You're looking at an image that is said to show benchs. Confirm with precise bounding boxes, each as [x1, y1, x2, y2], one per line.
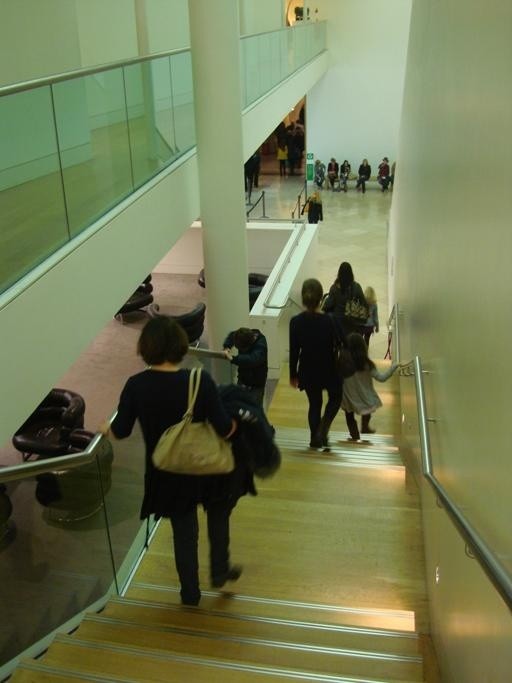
[315, 171, 393, 191]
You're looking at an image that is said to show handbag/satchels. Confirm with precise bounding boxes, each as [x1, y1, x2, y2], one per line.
[150, 367, 236, 475]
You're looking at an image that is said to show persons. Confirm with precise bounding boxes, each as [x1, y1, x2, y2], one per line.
[327, 158, 340, 191]
[362, 287, 379, 347]
[244, 157, 255, 197]
[300, 192, 323, 223]
[276, 121, 305, 176]
[340, 160, 351, 193]
[253, 153, 259, 188]
[314, 160, 326, 191]
[355, 159, 372, 194]
[96, 315, 243, 608]
[288, 278, 369, 448]
[341, 332, 401, 440]
[222, 327, 268, 407]
[324, 262, 368, 335]
[377, 157, 391, 193]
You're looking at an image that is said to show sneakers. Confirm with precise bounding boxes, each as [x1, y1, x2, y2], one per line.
[209, 565, 243, 589]
[360, 426, 376, 434]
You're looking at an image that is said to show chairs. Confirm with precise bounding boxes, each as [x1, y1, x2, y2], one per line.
[0, 387, 115, 552]
[118, 273, 269, 346]
